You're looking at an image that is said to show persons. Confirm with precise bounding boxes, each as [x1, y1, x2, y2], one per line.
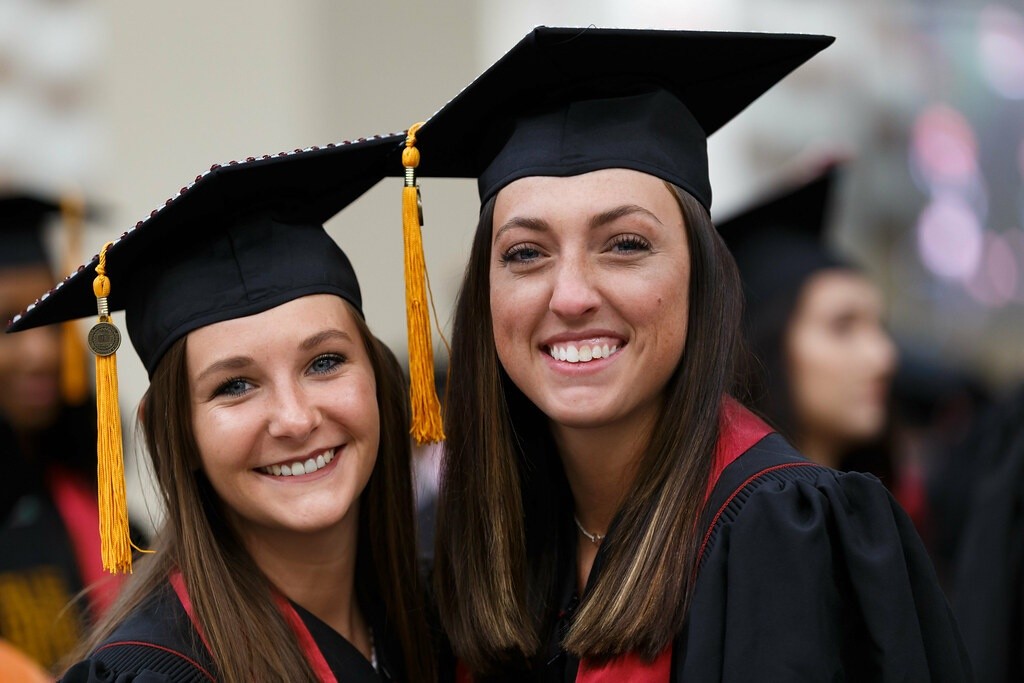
[1, 183, 154, 682]
[713, 160, 897, 470]
[383, 26, 976, 683]
[7, 129, 438, 683]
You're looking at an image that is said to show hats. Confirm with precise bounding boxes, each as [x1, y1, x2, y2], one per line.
[399, 24, 836, 451]
[711, 175, 854, 309]
[2, 191, 98, 407]
[4, 132, 409, 577]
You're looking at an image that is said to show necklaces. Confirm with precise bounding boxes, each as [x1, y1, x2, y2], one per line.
[569, 509, 617, 547]
[363, 616, 382, 677]
